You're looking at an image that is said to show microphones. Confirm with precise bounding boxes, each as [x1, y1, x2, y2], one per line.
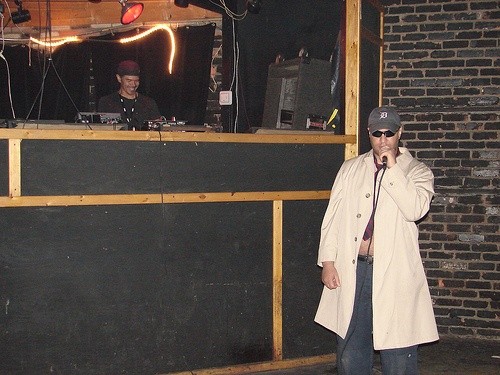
[381, 156, 388, 170]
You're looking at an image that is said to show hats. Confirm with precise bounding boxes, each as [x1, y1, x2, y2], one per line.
[368, 107, 401, 134]
[118, 60, 141, 75]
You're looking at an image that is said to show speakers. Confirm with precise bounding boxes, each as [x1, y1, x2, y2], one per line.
[261, 57, 333, 130]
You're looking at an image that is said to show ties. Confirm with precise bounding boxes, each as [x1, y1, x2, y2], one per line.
[363, 147, 401, 241]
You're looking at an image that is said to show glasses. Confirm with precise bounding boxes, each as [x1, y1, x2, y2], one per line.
[371, 130, 396, 138]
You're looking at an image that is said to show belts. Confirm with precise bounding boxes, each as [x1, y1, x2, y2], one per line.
[358, 255, 373, 265]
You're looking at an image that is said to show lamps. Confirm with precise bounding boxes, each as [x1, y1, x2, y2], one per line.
[11, 0, 31, 24]
[119, 0, 144, 25]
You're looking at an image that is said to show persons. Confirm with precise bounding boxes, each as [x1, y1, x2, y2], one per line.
[97, 61, 160, 131]
[314, 106, 439, 373]
